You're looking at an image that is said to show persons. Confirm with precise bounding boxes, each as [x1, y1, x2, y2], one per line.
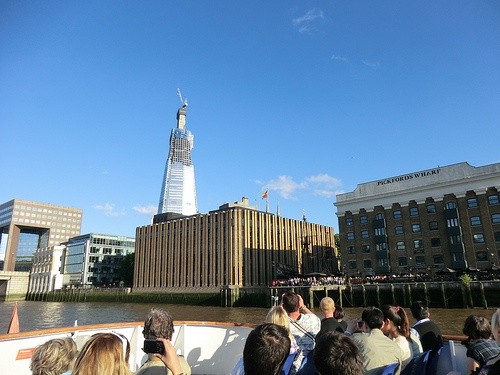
[28, 306, 191, 375]
[228, 290, 500, 375]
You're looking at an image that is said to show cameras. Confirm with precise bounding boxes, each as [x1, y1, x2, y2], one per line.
[144, 339, 164, 354]
